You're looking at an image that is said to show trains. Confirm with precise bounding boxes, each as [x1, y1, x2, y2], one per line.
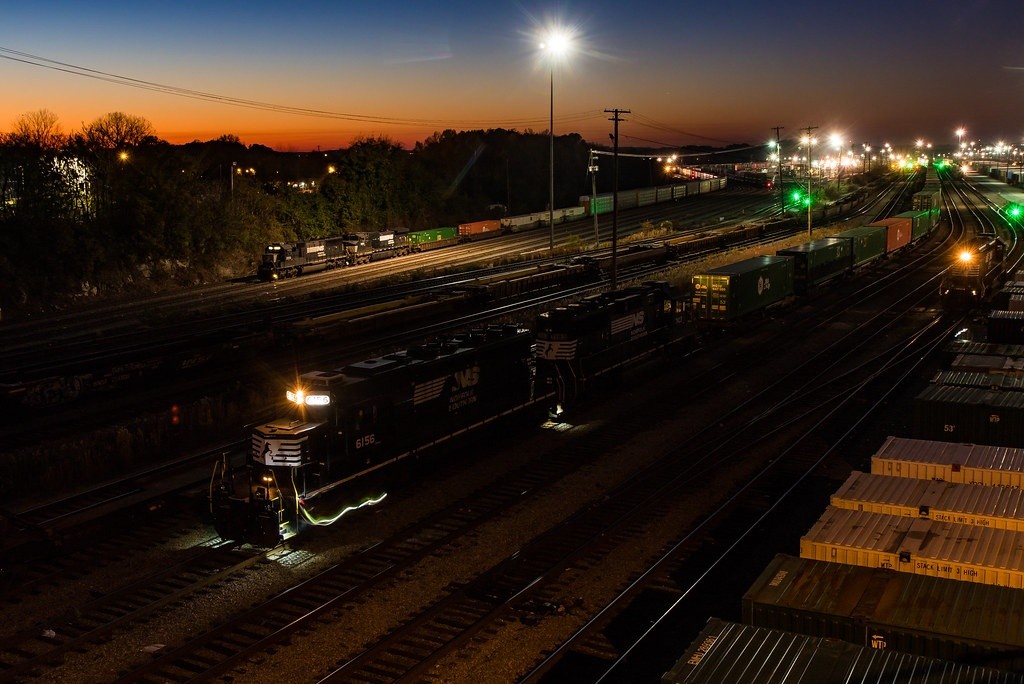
[255, 164, 731, 283]
[210, 163, 940, 526]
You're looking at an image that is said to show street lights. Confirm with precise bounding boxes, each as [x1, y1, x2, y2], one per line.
[533, 22, 1023, 253]
